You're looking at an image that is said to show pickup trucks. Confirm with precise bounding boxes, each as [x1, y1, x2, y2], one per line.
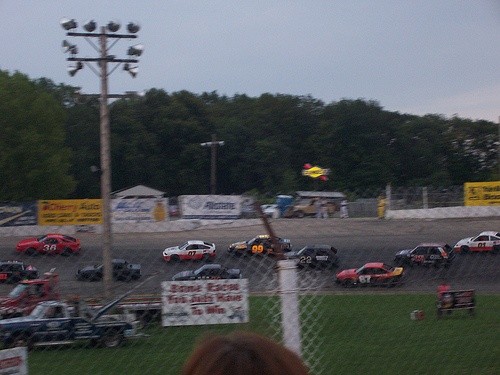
[0, 268, 81, 319]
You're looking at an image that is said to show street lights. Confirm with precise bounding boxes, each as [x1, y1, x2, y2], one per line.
[200, 140, 226, 195]
[59, 17, 145, 317]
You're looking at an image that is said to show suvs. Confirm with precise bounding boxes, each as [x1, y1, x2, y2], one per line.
[393, 242, 456, 269]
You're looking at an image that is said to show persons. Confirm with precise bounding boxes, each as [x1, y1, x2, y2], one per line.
[376, 194, 385, 218]
[182, 329, 312, 375]
[436, 281, 450, 301]
[339, 200, 348, 218]
[319, 199, 329, 218]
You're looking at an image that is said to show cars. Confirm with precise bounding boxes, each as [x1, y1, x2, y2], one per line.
[334, 262, 406, 289]
[282, 244, 339, 271]
[0, 299, 135, 352]
[171, 264, 243, 281]
[453, 230, 500, 256]
[289, 198, 335, 219]
[16, 233, 82, 257]
[163, 240, 217, 265]
[227, 234, 293, 260]
[75, 259, 143, 284]
[0, 259, 40, 284]
